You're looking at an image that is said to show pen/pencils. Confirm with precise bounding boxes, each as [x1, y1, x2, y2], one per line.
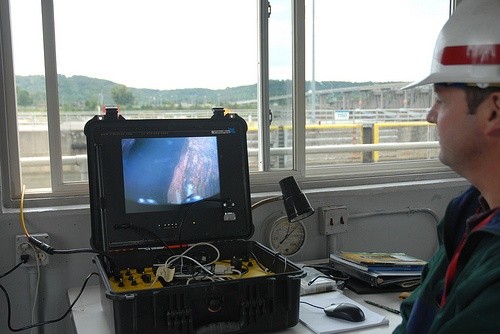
[363, 299, 399, 314]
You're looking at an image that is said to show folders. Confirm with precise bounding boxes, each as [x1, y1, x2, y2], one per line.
[329, 258, 426, 286]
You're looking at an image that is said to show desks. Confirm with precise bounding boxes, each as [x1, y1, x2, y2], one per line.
[67, 257, 408, 334]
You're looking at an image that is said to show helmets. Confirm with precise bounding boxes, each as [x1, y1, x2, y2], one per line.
[401, 0, 500, 93]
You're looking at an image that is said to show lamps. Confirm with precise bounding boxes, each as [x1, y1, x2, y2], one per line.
[251, 176, 316, 223]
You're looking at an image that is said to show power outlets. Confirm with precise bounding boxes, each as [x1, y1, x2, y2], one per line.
[16, 233, 51, 268]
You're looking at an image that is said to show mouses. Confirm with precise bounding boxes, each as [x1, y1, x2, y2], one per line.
[324, 303, 365, 322]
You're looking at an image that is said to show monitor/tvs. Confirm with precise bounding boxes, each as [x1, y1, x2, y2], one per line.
[83, 106, 254, 251]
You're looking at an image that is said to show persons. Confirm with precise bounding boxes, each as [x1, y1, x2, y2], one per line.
[392, 0, 500, 334]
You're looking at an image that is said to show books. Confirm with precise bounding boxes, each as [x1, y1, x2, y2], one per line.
[331, 249, 426, 271]
